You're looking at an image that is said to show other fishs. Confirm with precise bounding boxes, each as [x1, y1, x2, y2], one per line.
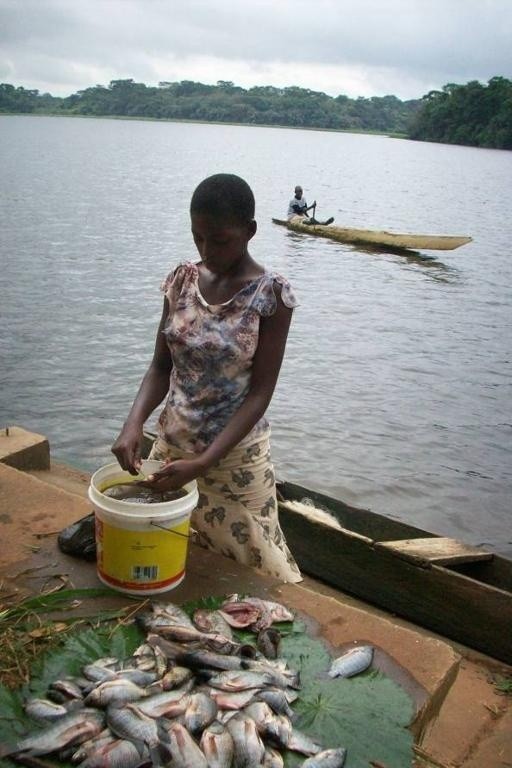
[99, 457, 188, 504]
[1, 592, 349, 767]
[325, 644, 375, 679]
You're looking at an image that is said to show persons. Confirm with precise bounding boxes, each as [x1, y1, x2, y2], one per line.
[111, 174, 303, 585]
[288, 185, 334, 226]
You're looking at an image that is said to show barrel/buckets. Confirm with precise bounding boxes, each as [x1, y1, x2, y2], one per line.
[86, 460, 200, 597]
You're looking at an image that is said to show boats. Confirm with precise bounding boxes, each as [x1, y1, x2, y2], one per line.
[273, 217, 473, 250]
[142, 433, 512, 661]
